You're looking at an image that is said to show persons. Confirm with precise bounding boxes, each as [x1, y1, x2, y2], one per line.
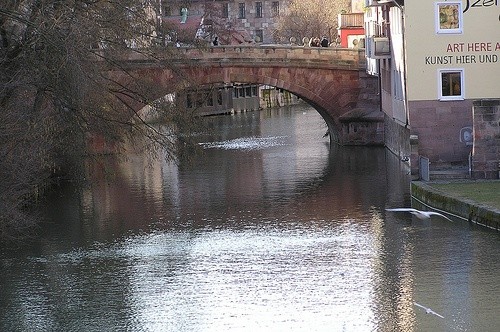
[336, 35, 342, 44]
[321, 36, 328, 47]
[310, 37, 321, 47]
[213, 37, 218, 45]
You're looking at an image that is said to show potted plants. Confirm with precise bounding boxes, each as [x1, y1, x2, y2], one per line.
[374, 33, 389, 53]
